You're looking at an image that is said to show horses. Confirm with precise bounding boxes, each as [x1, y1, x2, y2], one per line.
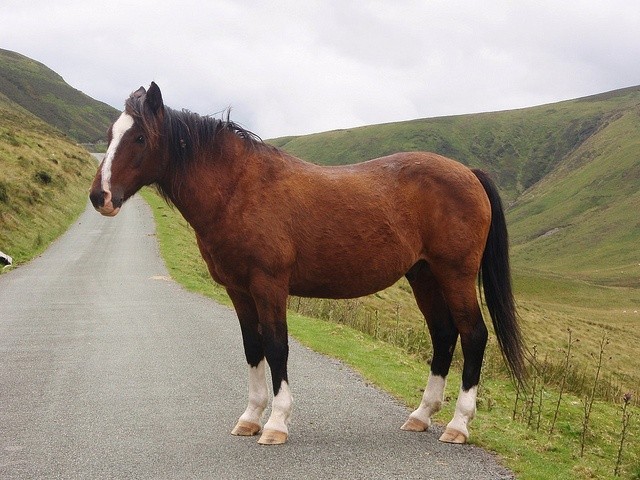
[86, 79, 544, 445]
